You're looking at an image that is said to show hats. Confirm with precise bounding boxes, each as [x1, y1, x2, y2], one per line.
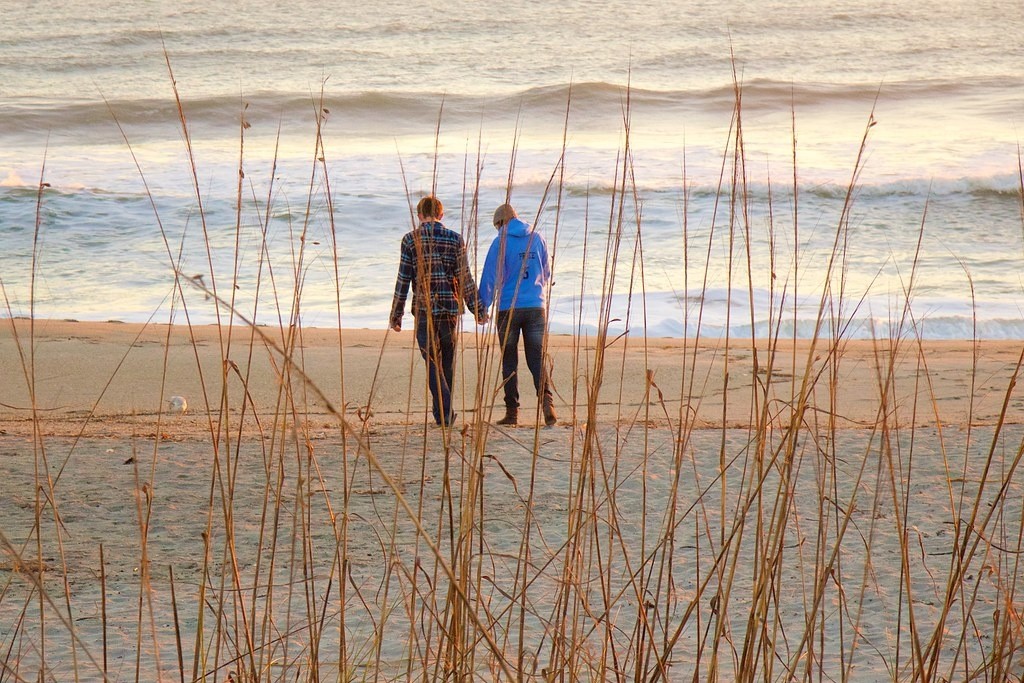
[493, 204, 517, 226]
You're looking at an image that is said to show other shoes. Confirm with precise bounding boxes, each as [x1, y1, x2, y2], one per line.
[436, 413, 450, 426]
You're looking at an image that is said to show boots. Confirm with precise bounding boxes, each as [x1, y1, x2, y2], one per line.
[496, 407, 519, 425]
[540, 392, 557, 425]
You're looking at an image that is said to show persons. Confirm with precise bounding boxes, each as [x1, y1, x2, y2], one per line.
[475, 205, 559, 425]
[389, 197, 489, 426]
